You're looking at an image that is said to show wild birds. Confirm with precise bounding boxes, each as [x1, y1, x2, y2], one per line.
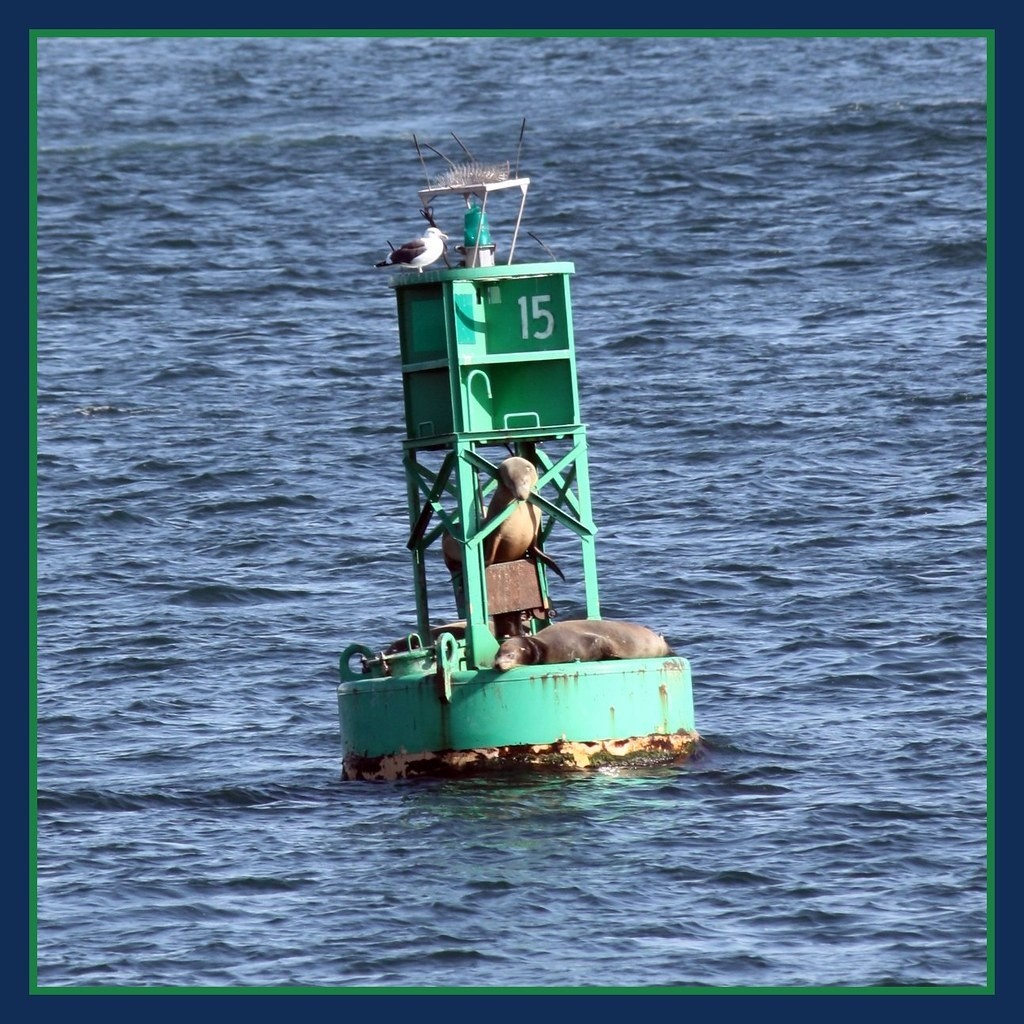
[373, 227, 448, 274]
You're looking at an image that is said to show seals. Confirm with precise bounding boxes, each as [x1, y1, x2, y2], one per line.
[494, 619, 674, 673]
[442, 456, 566, 583]
